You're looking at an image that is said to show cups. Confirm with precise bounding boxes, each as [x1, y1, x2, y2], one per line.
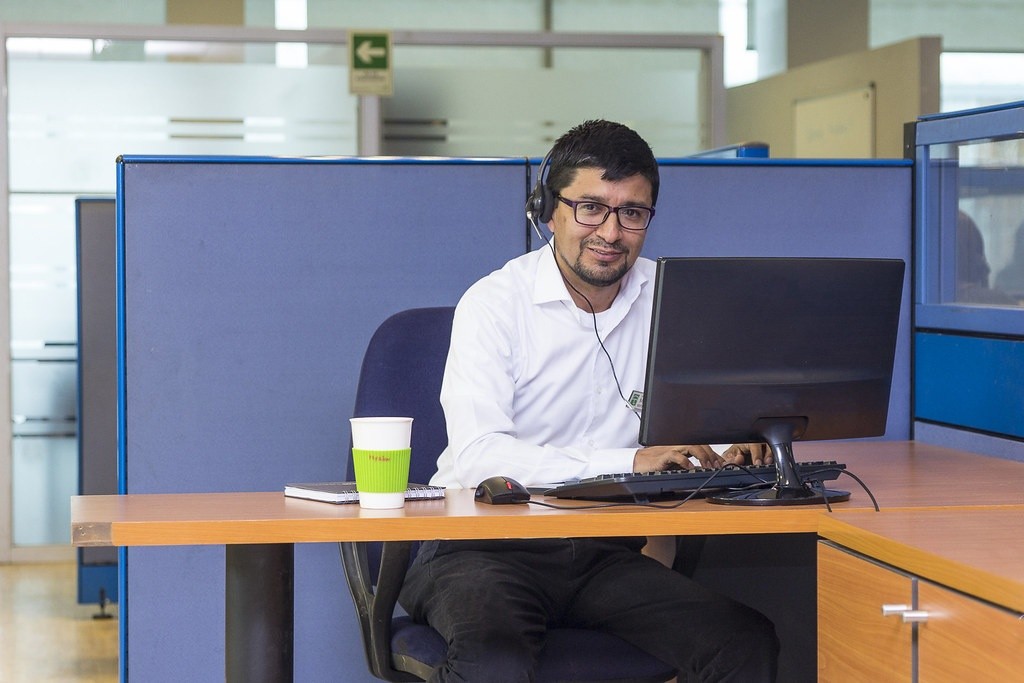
[349, 417, 414, 509]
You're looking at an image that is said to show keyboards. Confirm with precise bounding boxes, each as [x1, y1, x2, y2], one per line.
[544, 459, 846, 502]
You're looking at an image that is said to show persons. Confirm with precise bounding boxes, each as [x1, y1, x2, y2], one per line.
[955, 208, 1023, 304]
[396, 116, 774, 681]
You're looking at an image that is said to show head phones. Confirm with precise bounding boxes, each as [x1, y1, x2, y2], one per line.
[525, 149, 556, 239]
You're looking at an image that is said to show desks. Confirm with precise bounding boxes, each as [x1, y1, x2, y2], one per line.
[71, 440, 1024, 683]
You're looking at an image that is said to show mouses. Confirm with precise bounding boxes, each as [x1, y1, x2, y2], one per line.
[474, 476, 530, 505]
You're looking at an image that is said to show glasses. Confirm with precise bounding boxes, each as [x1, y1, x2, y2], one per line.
[557, 194, 655, 230]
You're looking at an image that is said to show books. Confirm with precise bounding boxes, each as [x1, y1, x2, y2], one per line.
[284, 479, 446, 506]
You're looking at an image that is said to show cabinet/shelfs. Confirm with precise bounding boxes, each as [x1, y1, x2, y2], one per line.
[817, 508, 1024, 682]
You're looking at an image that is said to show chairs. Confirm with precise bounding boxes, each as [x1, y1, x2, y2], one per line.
[340, 305, 459, 683]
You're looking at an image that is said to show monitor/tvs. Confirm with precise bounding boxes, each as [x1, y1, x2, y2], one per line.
[638, 258, 906, 505]
